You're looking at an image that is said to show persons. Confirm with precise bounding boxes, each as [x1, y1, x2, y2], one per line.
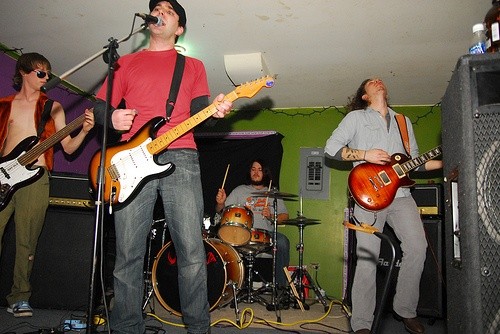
[0, 52, 95, 317]
[216, 160, 289, 301]
[323, 78, 442, 334]
[93, 0, 233, 334]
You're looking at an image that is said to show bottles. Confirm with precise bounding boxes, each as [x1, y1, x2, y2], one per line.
[484, 0, 500, 52]
[469, 23, 488, 55]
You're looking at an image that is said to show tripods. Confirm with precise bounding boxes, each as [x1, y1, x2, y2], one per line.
[241, 198, 330, 311]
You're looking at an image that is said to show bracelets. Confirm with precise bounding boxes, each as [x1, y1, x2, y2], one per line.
[364, 150, 367, 161]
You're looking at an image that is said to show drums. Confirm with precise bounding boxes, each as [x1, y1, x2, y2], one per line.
[151, 237, 245, 318]
[215, 203, 255, 247]
[248, 228, 273, 247]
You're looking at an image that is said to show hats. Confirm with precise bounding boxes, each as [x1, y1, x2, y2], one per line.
[149, 0, 186, 28]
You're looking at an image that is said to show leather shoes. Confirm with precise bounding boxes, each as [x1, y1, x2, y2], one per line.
[355, 329, 371, 334]
[393, 311, 425, 334]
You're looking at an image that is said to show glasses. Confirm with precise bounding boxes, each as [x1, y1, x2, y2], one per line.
[34, 70, 52, 78]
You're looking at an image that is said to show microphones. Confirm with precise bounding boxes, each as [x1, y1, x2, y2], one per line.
[135, 12, 163, 27]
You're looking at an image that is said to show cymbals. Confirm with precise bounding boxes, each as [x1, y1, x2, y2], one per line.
[279, 216, 322, 226]
[251, 191, 299, 202]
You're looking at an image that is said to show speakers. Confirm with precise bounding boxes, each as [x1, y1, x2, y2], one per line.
[0, 206, 108, 313]
[347, 217, 445, 320]
[440, 50, 500, 334]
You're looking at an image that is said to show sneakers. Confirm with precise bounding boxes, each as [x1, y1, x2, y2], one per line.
[7, 301, 33, 317]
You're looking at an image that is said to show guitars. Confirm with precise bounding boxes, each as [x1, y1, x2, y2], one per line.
[347, 144, 442, 214]
[0, 107, 95, 212]
[87, 74, 275, 212]
